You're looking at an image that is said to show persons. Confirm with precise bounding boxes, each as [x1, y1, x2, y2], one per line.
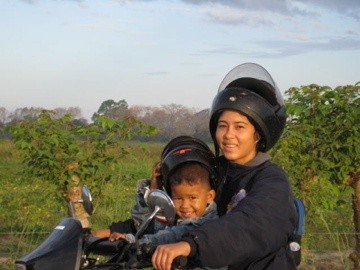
[108, 162, 228, 270]
[90, 102, 299, 270]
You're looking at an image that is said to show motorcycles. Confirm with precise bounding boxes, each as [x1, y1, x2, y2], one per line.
[15, 183, 177, 270]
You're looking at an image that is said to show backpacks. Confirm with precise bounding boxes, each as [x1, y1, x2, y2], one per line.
[289, 198, 304, 266]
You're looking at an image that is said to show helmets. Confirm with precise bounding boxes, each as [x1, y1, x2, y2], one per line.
[160, 136, 219, 196]
[209, 63, 286, 153]
